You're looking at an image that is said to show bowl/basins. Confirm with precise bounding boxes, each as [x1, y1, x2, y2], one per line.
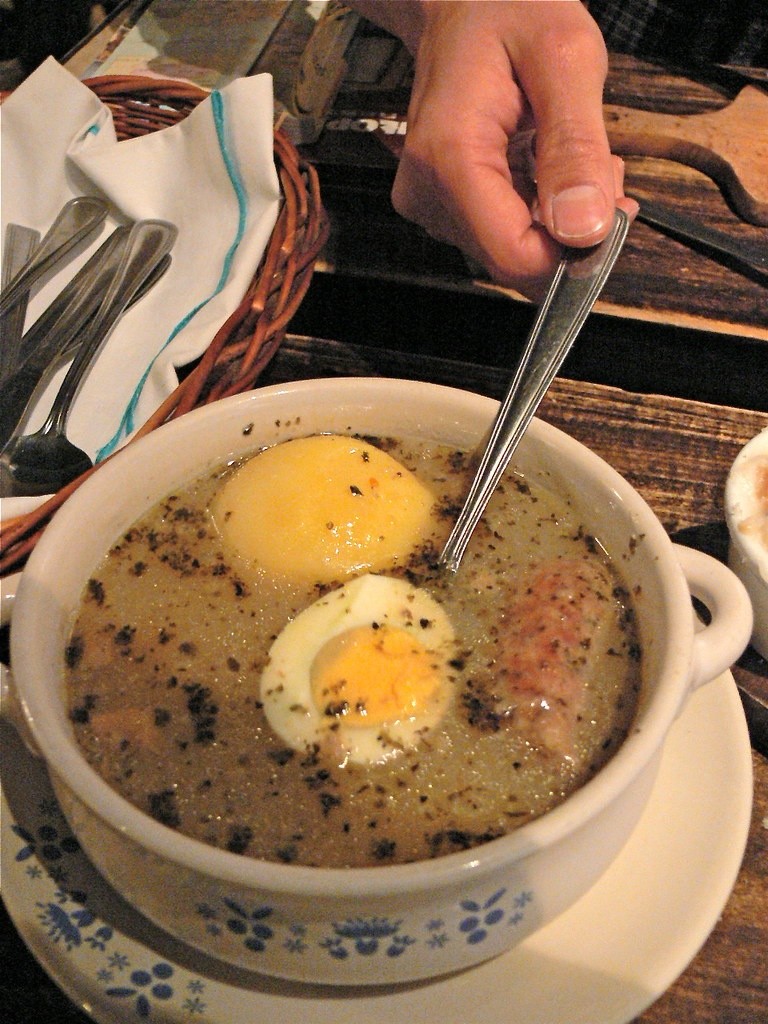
[0, 376, 754, 983]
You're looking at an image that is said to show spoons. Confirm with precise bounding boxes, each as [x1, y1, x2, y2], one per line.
[0, 219, 177, 500]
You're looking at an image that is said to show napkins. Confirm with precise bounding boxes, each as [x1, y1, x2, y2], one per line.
[0, 56, 283, 528]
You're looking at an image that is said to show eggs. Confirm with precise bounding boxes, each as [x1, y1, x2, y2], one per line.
[257, 570, 458, 768]
[207, 436, 442, 589]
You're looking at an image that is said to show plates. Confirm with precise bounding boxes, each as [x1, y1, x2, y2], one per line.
[0, 614, 752, 1024]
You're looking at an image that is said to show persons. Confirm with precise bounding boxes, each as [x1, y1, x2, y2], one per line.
[351, 1, 768, 310]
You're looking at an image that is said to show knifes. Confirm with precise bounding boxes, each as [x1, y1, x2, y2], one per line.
[0, 226, 137, 456]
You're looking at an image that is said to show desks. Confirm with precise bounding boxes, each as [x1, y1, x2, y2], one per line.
[0, 0, 768, 1024]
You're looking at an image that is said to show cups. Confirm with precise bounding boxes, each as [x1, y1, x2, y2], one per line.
[724, 428, 767, 661]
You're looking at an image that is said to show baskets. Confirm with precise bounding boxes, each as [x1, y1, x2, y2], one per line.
[0, 74, 331, 580]
